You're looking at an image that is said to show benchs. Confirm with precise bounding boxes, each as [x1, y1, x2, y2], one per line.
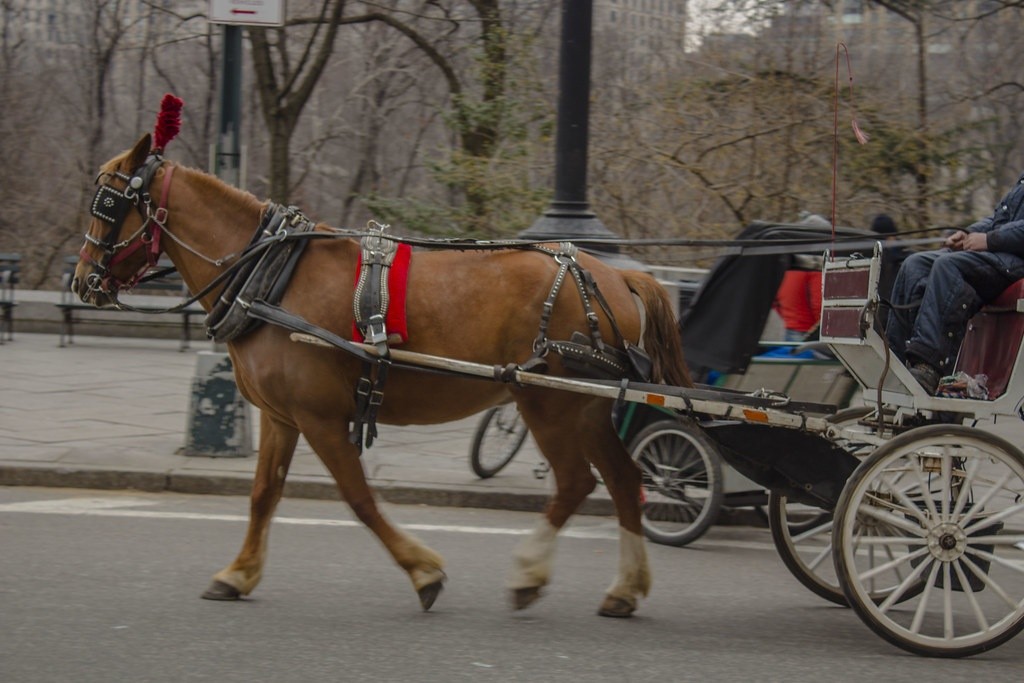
[952, 277, 1024, 399]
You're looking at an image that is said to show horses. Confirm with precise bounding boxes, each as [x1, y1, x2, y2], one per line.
[69, 133, 710, 619]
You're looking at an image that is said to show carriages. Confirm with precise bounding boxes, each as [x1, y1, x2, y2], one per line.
[70, 94, 1024, 661]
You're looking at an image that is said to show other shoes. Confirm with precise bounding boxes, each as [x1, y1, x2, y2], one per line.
[908, 362, 940, 397]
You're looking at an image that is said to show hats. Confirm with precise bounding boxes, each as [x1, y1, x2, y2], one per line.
[873, 214, 898, 236]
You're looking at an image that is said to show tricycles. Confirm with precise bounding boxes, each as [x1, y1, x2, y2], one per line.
[470, 394, 834, 545]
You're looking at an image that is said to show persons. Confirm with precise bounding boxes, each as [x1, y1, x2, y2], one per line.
[882, 173, 1024, 396]
[869, 213, 898, 240]
